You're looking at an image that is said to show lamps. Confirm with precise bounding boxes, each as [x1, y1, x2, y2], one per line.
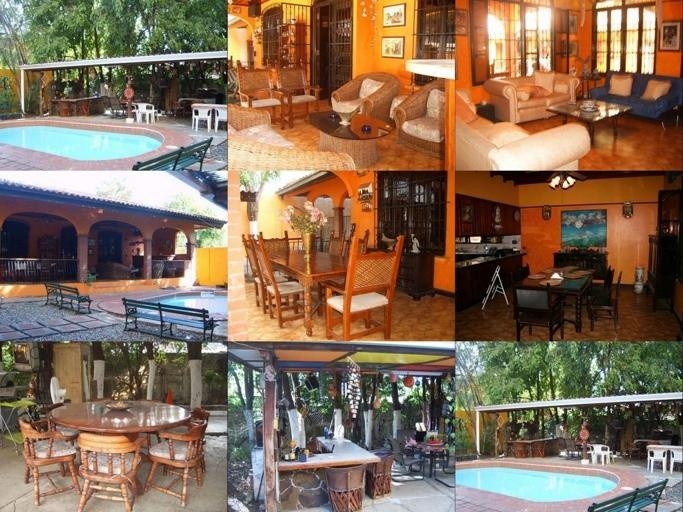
[548, 175, 579, 192]
[541, 204, 552, 222]
[622, 199, 633, 219]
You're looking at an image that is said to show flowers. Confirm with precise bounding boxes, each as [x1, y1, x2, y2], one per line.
[281, 199, 330, 237]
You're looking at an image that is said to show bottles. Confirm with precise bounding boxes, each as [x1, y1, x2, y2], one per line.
[327, 429, 332, 444]
[323, 424, 329, 444]
[94, 90, 98, 99]
[291, 19, 296, 25]
[581, 64, 587, 80]
[283, 446, 299, 461]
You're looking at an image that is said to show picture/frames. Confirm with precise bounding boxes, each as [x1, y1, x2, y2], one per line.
[381, 2, 406, 28]
[657, 21, 682, 54]
[380, 35, 405, 60]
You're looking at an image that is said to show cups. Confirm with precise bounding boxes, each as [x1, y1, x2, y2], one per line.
[276, 19, 280, 25]
[286, 19, 290, 24]
[299, 453, 305, 462]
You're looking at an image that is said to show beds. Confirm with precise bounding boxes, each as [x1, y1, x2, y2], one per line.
[302, 108, 394, 178]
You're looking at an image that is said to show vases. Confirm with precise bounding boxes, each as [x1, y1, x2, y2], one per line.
[300, 228, 316, 260]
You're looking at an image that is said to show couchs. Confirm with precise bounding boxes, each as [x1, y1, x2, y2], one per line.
[227, 101, 356, 173]
[590, 68, 682, 131]
[482, 69, 582, 126]
[455, 89, 593, 174]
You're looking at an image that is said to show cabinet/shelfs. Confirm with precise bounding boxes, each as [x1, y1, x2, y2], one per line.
[259, 6, 308, 77]
[455, 190, 522, 241]
[378, 243, 437, 303]
[644, 230, 683, 314]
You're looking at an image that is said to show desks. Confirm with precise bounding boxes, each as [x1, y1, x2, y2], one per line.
[274, 431, 383, 471]
[506, 435, 556, 458]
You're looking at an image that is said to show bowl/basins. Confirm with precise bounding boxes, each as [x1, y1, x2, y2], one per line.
[581, 101, 593, 110]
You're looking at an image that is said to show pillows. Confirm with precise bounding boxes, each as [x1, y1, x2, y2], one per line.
[638, 78, 671, 102]
[424, 86, 447, 120]
[607, 72, 635, 98]
[514, 71, 569, 102]
[356, 77, 386, 101]
[454, 91, 481, 125]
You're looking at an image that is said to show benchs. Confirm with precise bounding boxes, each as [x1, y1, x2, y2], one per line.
[41, 279, 95, 315]
[116, 297, 219, 344]
[129, 136, 214, 172]
[586, 478, 669, 512]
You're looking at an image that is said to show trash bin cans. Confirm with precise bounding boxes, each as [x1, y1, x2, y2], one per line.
[326, 464, 364, 512]
[367, 450, 394, 500]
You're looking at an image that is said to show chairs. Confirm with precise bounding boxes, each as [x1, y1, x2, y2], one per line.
[0, 394, 211, 512]
[103, 89, 227, 134]
[271, 56, 325, 130]
[329, 70, 404, 134]
[383, 424, 455, 489]
[507, 257, 624, 342]
[583, 440, 683, 475]
[389, 76, 447, 161]
[237, 221, 409, 343]
[234, 58, 288, 133]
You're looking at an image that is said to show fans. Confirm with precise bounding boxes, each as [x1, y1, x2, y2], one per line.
[544, 170, 590, 184]
[48, 374, 67, 405]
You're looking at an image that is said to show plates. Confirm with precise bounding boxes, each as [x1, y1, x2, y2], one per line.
[525, 265, 590, 287]
[104, 401, 134, 411]
[425, 442, 443, 446]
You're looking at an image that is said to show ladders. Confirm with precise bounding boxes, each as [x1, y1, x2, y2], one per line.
[481, 265, 510, 309]
